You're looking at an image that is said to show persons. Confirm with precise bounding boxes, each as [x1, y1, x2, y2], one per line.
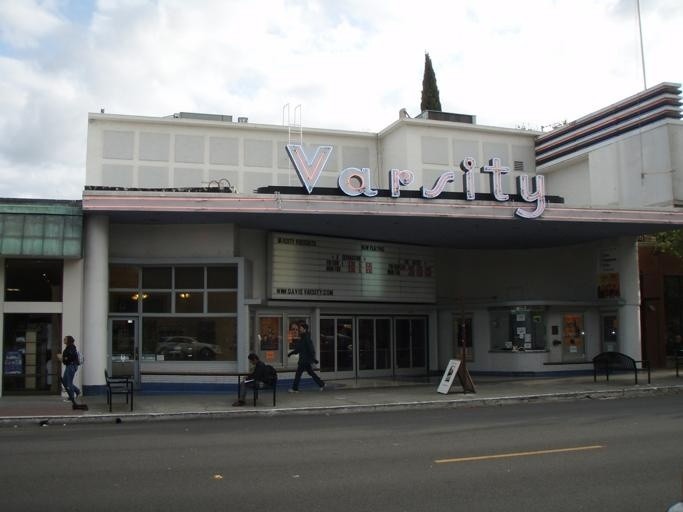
[57, 335, 82, 403]
[287, 322, 327, 395]
[232, 353, 273, 407]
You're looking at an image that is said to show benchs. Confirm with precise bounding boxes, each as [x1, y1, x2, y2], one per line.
[593, 352, 651, 384]
[238, 373, 277, 407]
[105, 369, 134, 412]
[674, 347, 683, 377]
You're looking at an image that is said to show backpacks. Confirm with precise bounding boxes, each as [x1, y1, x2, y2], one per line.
[77, 351, 84, 365]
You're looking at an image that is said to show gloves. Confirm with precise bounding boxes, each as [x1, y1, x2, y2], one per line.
[287, 352, 292, 357]
[313, 360, 318, 364]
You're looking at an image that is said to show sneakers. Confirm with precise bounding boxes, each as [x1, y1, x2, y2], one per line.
[319, 383, 326, 392]
[76, 391, 81, 400]
[63, 398, 73, 402]
[287, 388, 298, 393]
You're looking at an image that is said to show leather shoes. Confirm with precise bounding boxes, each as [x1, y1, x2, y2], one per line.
[232, 402, 244, 406]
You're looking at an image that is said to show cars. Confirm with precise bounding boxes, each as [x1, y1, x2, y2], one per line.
[273, 326, 351, 356]
[154, 333, 224, 362]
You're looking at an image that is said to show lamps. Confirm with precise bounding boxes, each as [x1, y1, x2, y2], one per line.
[132, 292, 149, 300]
[179, 292, 192, 304]
